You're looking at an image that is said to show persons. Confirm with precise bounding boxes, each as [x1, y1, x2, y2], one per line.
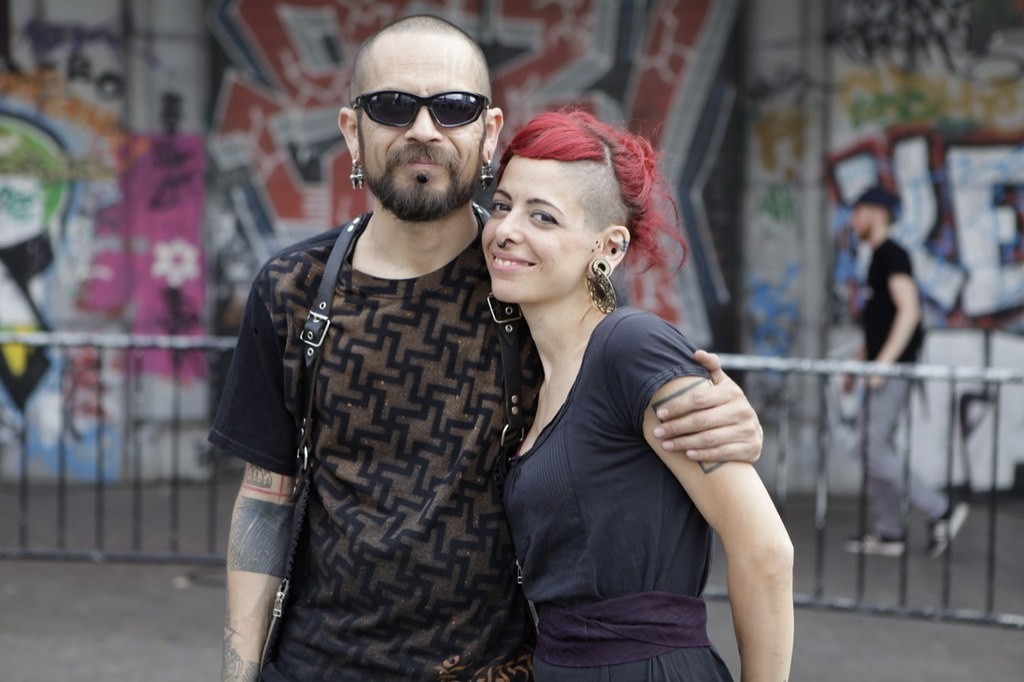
[481, 112, 797, 682]
[840, 184, 968, 560]
[207, 16, 765, 680]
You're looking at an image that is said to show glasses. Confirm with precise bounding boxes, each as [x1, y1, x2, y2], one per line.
[349, 90, 490, 129]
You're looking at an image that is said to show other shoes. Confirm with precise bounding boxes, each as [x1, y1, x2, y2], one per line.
[846, 527, 906, 556]
[925, 498, 967, 562]
